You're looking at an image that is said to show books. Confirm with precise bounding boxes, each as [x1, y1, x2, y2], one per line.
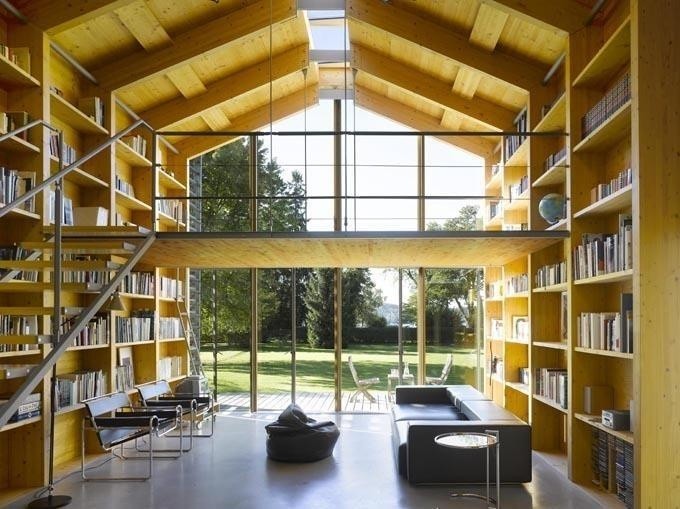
[533, 368, 567, 409]
[2, 111, 28, 141]
[2, 393, 41, 421]
[543, 148, 566, 174]
[116, 347, 135, 390]
[560, 292, 567, 343]
[50, 133, 75, 164]
[159, 277, 181, 380]
[116, 213, 137, 227]
[2, 314, 39, 352]
[55, 368, 106, 412]
[573, 213, 631, 281]
[581, 75, 630, 139]
[115, 310, 155, 343]
[534, 262, 567, 287]
[116, 176, 134, 198]
[159, 148, 182, 222]
[74, 206, 108, 226]
[62, 253, 108, 283]
[50, 190, 73, 225]
[488, 273, 529, 387]
[119, 133, 146, 157]
[489, 113, 528, 231]
[2, 246, 39, 282]
[589, 427, 631, 506]
[2, 44, 30, 76]
[117, 271, 154, 295]
[79, 97, 106, 129]
[589, 168, 631, 205]
[2, 167, 35, 213]
[51, 313, 109, 346]
[577, 293, 630, 353]
[583, 385, 613, 414]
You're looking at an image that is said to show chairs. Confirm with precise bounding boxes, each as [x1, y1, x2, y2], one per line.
[348, 356, 380, 402]
[425, 353, 454, 385]
[134, 380, 214, 452]
[81, 390, 182, 481]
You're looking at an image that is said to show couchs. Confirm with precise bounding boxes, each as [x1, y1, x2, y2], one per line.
[390, 385, 532, 486]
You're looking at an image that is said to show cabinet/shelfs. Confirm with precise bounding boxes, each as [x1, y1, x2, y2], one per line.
[434, 430, 500, 509]
[568, 1, 680, 508]
[0, 2, 48, 503]
[528, 38, 569, 481]
[501, 105, 530, 447]
[195, 402, 220, 422]
[485, 139, 502, 411]
[157, 135, 190, 397]
[48, 36, 115, 488]
[115, 96, 158, 449]
